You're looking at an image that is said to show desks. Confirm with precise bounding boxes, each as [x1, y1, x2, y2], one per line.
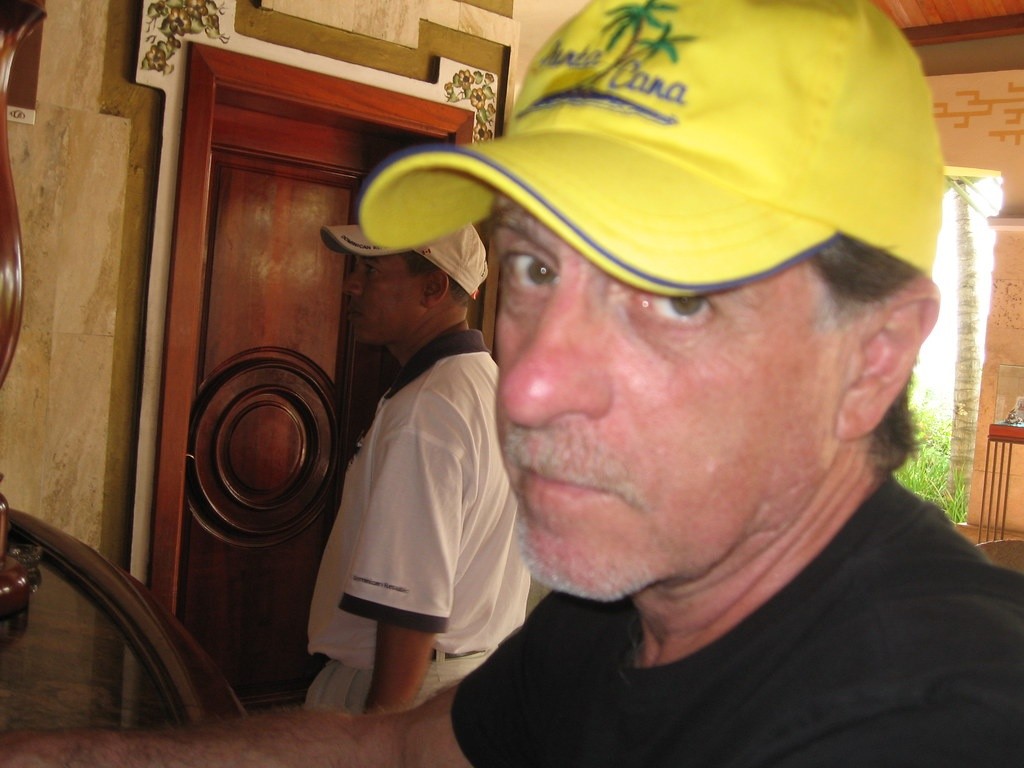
[979, 435, 1024, 543]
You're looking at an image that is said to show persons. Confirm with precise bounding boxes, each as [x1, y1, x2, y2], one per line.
[0, 0, 1024, 768]
[308, 216, 532, 720]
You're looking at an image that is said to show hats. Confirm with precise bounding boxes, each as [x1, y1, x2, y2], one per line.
[320, 215, 488, 300]
[354, 0, 944, 297]
